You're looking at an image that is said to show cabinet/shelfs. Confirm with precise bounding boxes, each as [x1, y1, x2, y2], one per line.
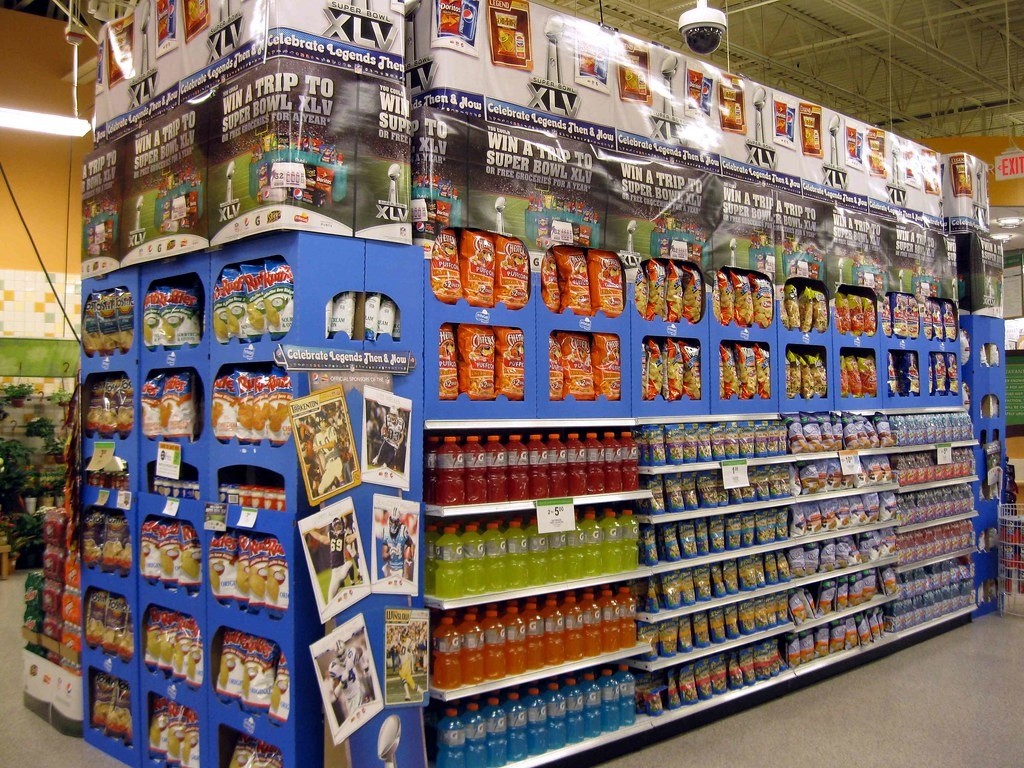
[81, 231, 427, 768]
[960, 314, 1006, 619]
[425, 257, 979, 768]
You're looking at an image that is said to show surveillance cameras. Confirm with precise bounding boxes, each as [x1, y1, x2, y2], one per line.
[678, 7, 727, 57]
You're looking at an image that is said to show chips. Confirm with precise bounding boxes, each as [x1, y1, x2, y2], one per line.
[80, 297, 289, 768]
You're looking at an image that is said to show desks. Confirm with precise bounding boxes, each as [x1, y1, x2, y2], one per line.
[0, 545, 11, 580]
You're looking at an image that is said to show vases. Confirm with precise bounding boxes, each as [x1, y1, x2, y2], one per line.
[56, 496, 64, 507]
[0, 536, 8, 546]
[41, 497, 54, 507]
[25, 498, 36, 515]
[8, 558, 16, 574]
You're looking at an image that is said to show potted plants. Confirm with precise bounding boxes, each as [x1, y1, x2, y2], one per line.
[3, 384, 33, 407]
[52, 390, 73, 407]
[25, 417, 67, 464]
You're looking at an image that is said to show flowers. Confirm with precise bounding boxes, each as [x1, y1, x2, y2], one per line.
[0, 464, 67, 574]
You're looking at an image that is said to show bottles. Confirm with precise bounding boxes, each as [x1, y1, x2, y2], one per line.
[424, 508, 643, 768]
[423, 429, 637, 507]
